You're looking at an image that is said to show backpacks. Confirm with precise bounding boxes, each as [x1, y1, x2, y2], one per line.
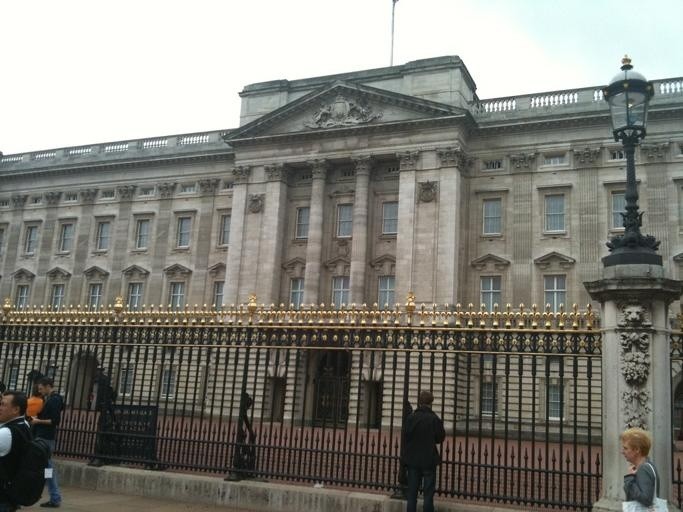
[4, 423, 50, 507]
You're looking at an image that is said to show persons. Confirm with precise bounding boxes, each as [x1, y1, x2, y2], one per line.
[400, 391, 445, 512]
[620, 428, 660, 512]
[1, 377, 62, 511]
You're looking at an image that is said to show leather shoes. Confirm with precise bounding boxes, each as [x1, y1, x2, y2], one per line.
[41, 500, 60, 507]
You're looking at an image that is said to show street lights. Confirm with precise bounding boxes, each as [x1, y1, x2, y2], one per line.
[601, 53, 667, 269]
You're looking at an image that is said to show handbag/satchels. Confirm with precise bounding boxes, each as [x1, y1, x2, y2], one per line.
[622, 463, 669, 512]
